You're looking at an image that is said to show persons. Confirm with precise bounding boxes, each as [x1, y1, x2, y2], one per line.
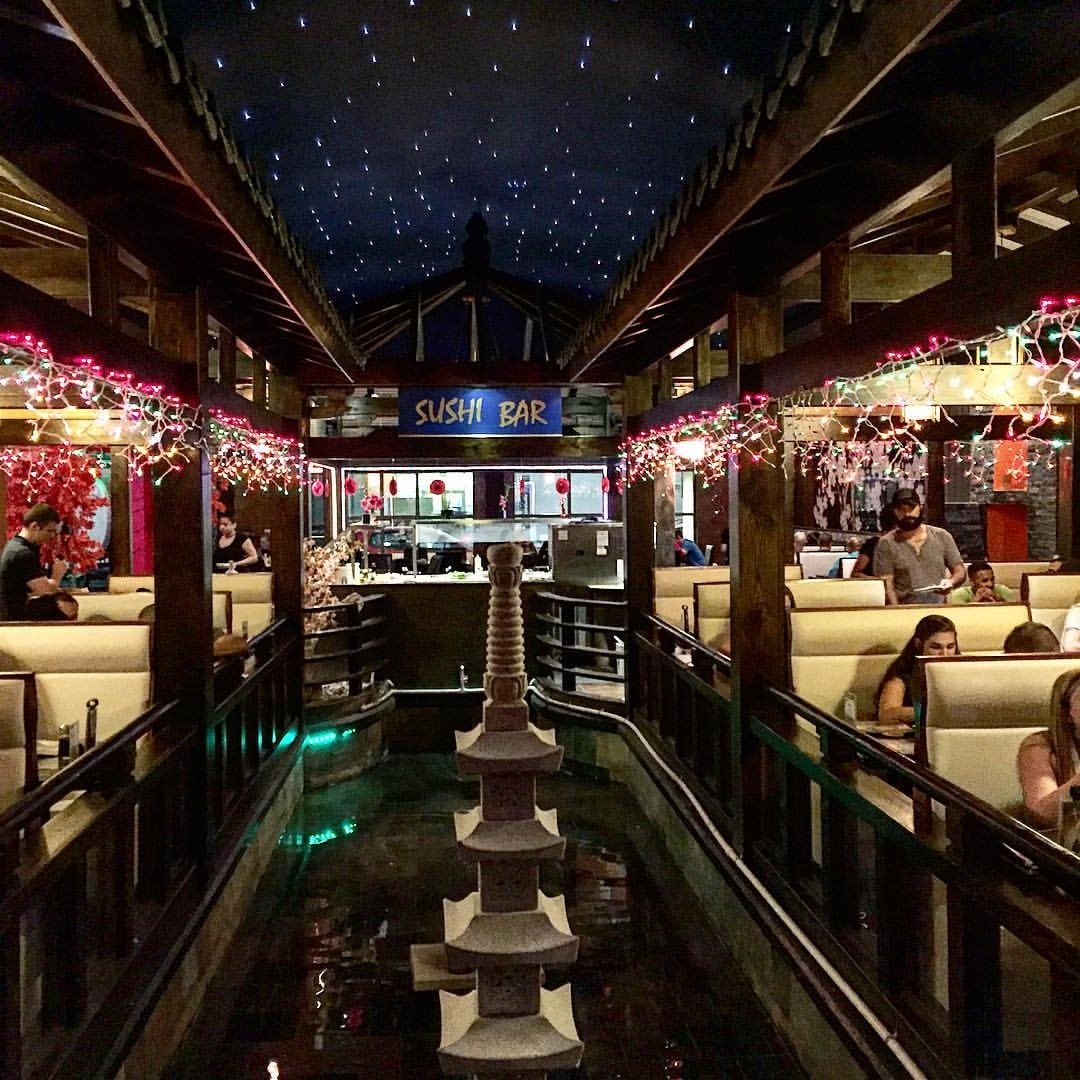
[1003, 621, 1061, 652]
[674, 529, 705, 565]
[948, 562, 1012, 603]
[429, 544, 474, 573]
[783, 585, 796, 608]
[212, 510, 271, 574]
[851, 506, 898, 579]
[873, 616, 963, 721]
[1060, 604, 1080, 651]
[0, 503, 67, 619]
[720, 528, 729, 561]
[1048, 557, 1080, 573]
[794, 530, 833, 553]
[1019, 671, 1080, 828]
[873, 490, 966, 607]
[519, 541, 548, 569]
[829, 539, 862, 577]
[139, 605, 154, 623]
[28, 592, 78, 620]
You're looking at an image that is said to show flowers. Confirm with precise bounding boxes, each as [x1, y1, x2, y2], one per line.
[360, 495, 384, 515]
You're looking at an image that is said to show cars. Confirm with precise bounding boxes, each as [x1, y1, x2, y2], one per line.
[322, 522, 469, 574]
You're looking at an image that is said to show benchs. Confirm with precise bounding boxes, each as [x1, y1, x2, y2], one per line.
[654, 562, 1080, 823]
[0, 572, 273, 812]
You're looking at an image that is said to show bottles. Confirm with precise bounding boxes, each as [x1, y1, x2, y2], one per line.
[58, 726, 69, 760]
[475, 554, 483, 579]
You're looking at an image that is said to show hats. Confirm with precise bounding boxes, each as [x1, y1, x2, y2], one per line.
[892, 489, 919, 505]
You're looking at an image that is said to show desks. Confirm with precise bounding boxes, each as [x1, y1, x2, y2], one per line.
[410, 515, 593, 577]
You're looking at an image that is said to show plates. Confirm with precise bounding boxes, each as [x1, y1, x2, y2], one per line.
[865, 725, 915, 738]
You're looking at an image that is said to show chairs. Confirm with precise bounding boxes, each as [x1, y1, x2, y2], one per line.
[351, 524, 417, 575]
[704, 545, 715, 566]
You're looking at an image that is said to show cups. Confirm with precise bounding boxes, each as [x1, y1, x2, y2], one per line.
[915, 702, 921, 737]
